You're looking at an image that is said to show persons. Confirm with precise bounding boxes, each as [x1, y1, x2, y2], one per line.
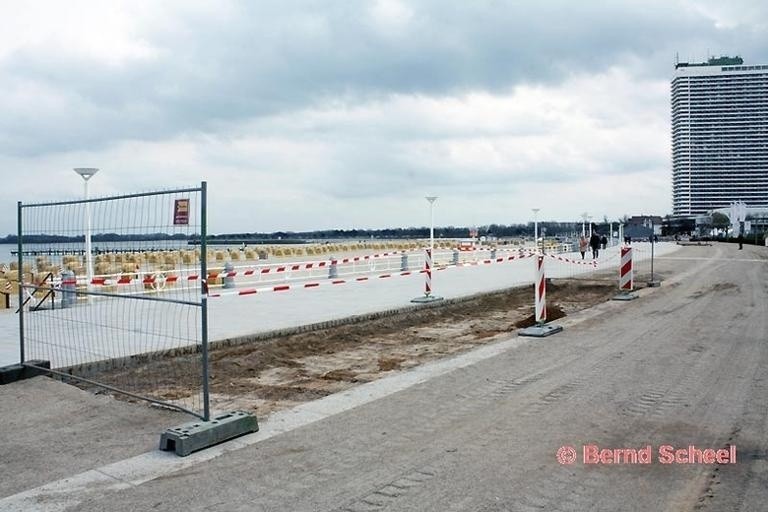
[624, 234, 632, 245]
[578, 236, 588, 260]
[588, 232, 601, 260]
[736, 232, 743, 249]
[649, 233, 658, 243]
[600, 234, 607, 249]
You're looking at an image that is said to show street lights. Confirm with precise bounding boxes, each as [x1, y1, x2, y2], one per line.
[587, 216, 593, 238]
[424, 196, 440, 260]
[532, 208, 540, 249]
[72, 167, 100, 284]
[609, 219, 613, 244]
[581, 215, 587, 237]
